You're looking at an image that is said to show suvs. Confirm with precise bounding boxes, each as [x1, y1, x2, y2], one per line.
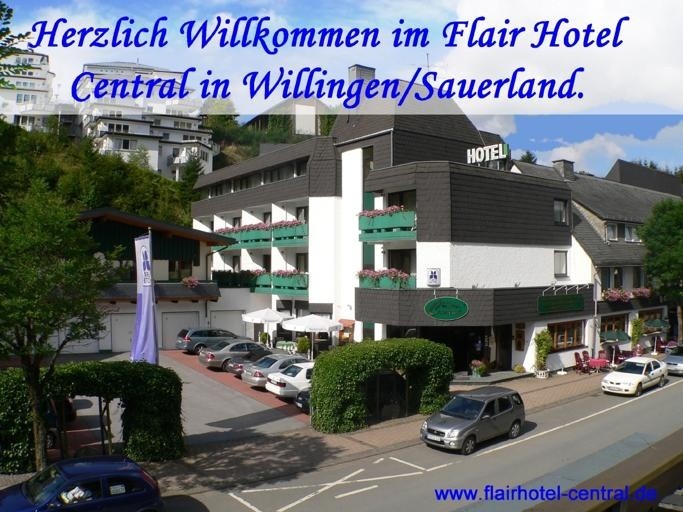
[175, 327, 252, 354]
[420, 385, 526, 455]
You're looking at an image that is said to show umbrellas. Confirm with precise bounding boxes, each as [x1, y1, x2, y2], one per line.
[601, 329, 631, 364]
[281, 315, 343, 361]
[242, 309, 294, 348]
[645, 318, 672, 352]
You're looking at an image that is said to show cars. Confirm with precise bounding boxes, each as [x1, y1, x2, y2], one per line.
[197, 339, 289, 372]
[226, 349, 290, 376]
[601, 357, 668, 397]
[241, 353, 314, 388]
[0, 455, 163, 512]
[661, 344, 683, 375]
[265, 361, 315, 400]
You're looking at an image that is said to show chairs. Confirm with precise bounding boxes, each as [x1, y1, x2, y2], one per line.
[582, 351, 590, 362]
[575, 352, 590, 375]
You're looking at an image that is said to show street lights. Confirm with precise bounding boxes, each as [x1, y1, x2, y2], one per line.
[593, 270, 600, 358]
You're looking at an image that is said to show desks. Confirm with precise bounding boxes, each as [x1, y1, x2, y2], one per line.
[586, 359, 610, 373]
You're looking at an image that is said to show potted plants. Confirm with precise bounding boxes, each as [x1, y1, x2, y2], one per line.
[535, 331, 553, 380]
